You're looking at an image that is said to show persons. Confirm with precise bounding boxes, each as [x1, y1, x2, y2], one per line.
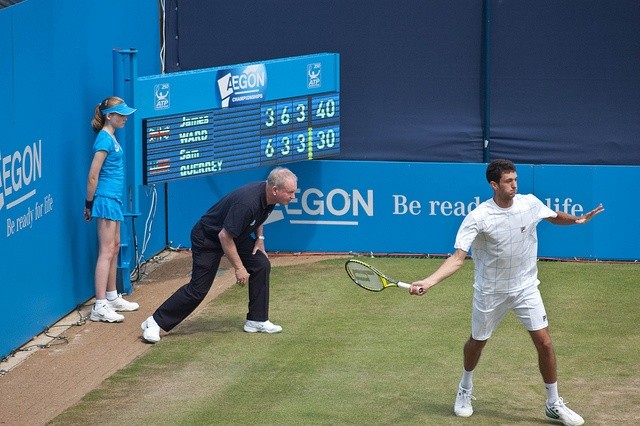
[407, 158, 604, 426]
[83, 96, 140, 322]
[140, 167, 298, 343]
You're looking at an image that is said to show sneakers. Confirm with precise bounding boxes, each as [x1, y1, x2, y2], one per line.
[106, 295, 139, 312]
[454, 379, 475, 416]
[545, 397, 584, 426]
[90, 303, 124, 322]
[141, 314, 160, 343]
[242, 319, 283, 333]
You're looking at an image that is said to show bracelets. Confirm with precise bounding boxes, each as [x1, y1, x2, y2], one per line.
[86, 200, 93, 209]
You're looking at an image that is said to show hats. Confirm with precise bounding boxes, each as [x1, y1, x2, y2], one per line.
[101, 102, 135, 116]
